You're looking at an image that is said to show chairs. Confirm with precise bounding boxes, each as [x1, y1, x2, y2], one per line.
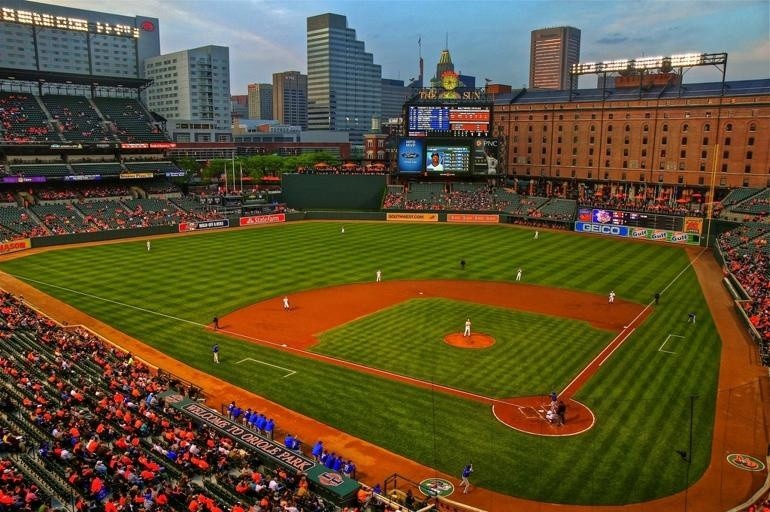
[1, 90, 576, 512]
[716, 185, 769, 357]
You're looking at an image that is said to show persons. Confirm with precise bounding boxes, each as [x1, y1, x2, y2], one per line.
[655, 293, 660, 305]
[1, 87, 294, 243]
[147, 240, 150, 251]
[465, 319, 471, 337]
[460, 464, 474, 494]
[609, 290, 615, 304]
[298, 161, 386, 176]
[461, 261, 465, 270]
[383, 179, 576, 224]
[376, 270, 381, 282]
[515, 266, 522, 281]
[0, 297, 433, 511]
[578, 181, 723, 219]
[534, 231, 539, 239]
[688, 312, 695, 324]
[547, 393, 565, 425]
[718, 198, 770, 365]
